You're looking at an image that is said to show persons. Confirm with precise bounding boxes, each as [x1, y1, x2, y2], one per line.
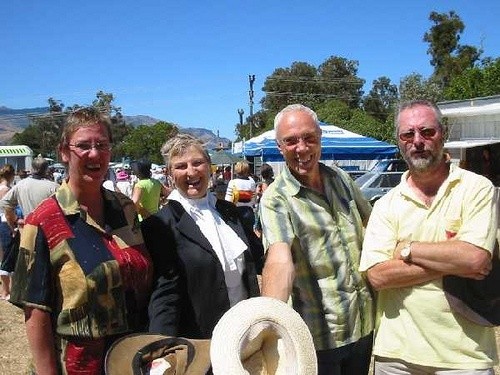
[141, 135, 270, 375]
[13, 161, 274, 221]
[0, 163, 18, 301]
[1, 157, 60, 238]
[358, 99, 500, 375]
[223, 160, 256, 213]
[132, 159, 172, 226]
[7, 106, 154, 374]
[259, 103, 379, 375]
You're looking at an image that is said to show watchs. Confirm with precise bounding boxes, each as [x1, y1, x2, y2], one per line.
[400, 239, 414, 263]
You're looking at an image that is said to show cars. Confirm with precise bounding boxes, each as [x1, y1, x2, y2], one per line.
[354, 171, 413, 207]
[0, 144, 169, 188]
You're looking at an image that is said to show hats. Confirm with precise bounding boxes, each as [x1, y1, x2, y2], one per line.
[442, 238, 500, 327]
[209, 296, 318, 375]
[104, 332, 211, 375]
[132, 159, 151, 174]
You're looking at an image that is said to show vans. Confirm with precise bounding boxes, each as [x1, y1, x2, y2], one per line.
[353, 159, 411, 188]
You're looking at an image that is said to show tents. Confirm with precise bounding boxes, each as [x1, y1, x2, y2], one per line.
[224, 119, 399, 182]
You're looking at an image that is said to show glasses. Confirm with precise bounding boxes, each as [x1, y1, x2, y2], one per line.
[66, 142, 113, 152]
[398, 128, 442, 141]
